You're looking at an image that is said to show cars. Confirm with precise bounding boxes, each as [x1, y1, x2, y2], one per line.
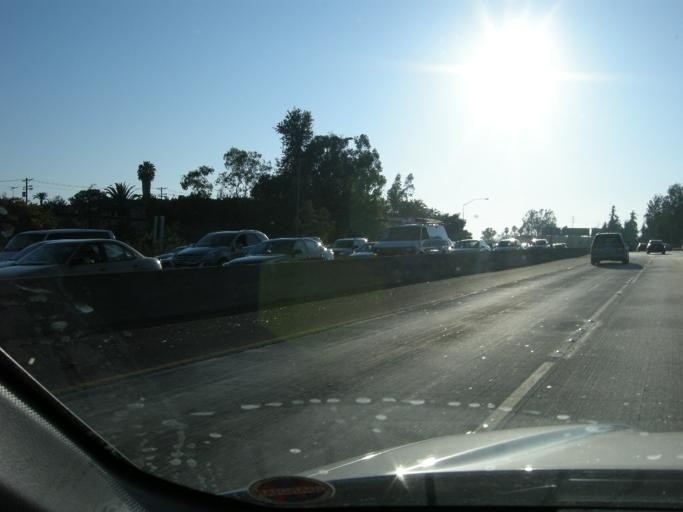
[493, 237, 568, 253]
[637, 240, 671, 255]
[453, 239, 490, 251]
[350, 241, 377, 255]
[222, 237, 335, 268]
[0, 238, 162, 280]
[591, 232, 629, 266]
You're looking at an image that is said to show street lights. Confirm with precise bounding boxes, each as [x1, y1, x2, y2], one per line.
[462, 197, 489, 220]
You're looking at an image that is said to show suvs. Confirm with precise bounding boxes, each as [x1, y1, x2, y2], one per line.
[374, 223, 452, 255]
[328, 237, 368, 255]
[167, 230, 270, 268]
[0, 229, 116, 268]
